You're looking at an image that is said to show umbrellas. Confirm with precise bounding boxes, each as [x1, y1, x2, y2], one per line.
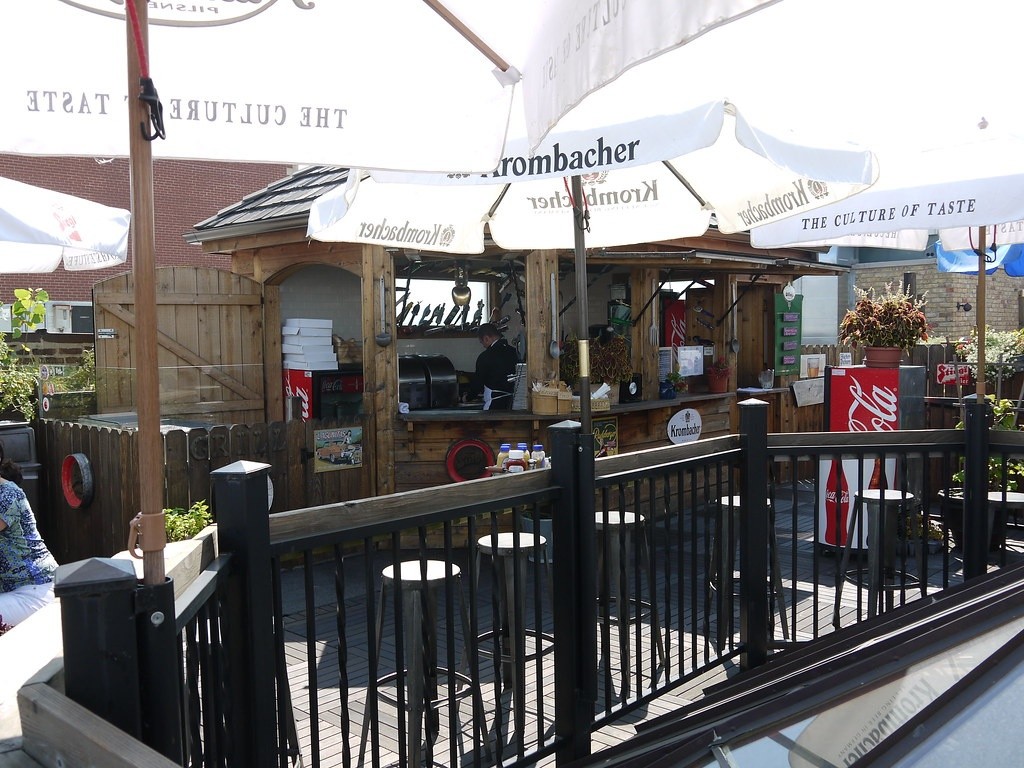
[0, 0, 1024, 436]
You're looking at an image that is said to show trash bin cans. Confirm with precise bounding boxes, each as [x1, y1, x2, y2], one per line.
[0, 427, 41, 524]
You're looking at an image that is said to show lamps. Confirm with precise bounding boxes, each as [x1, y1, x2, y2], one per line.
[451, 263, 471, 305]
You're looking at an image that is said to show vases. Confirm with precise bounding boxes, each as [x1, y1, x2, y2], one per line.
[863, 346, 903, 368]
[658, 381, 677, 400]
[707, 375, 728, 393]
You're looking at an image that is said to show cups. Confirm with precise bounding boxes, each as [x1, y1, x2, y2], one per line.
[531, 390, 572, 415]
[840, 353, 850, 366]
[807, 358, 820, 377]
[758, 369, 774, 388]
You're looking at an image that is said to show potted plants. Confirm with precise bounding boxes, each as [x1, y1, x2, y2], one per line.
[938, 393, 1016, 552]
[561, 333, 633, 404]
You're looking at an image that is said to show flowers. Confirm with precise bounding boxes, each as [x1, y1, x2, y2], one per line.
[835, 280, 935, 357]
[668, 363, 688, 395]
[707, 356, 732, 378]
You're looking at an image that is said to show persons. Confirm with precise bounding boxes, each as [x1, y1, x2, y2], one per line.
[0, 441, 60, 637]
[472, 324, 524, 412]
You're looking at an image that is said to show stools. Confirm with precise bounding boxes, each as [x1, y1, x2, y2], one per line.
[454, 533, 554, 755]
[595, 510, 667, 696]
[831, 490, 924, 631]
[357, 561, 495, 768]
[708, 495, 789, 651]
[984, 492, 1024, 562]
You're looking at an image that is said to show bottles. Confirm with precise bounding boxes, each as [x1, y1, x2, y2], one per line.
[499, 443, 545, 473]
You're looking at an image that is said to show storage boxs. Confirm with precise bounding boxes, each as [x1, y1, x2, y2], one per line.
[280, 319, 338, 370]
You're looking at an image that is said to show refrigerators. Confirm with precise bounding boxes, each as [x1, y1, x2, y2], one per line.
[607, 299, 684, 358]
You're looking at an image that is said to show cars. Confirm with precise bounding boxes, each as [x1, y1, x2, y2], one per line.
[316, 440, 347, 463]
